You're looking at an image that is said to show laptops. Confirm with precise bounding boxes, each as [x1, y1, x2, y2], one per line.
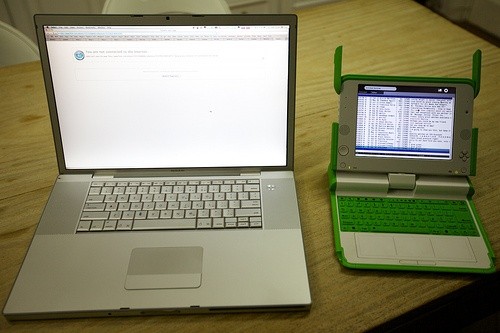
[330, 45, 495, 270]
[3, 12, 313, 313]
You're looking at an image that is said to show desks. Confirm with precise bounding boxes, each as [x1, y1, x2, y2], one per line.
[0, 0, 500, 333]
